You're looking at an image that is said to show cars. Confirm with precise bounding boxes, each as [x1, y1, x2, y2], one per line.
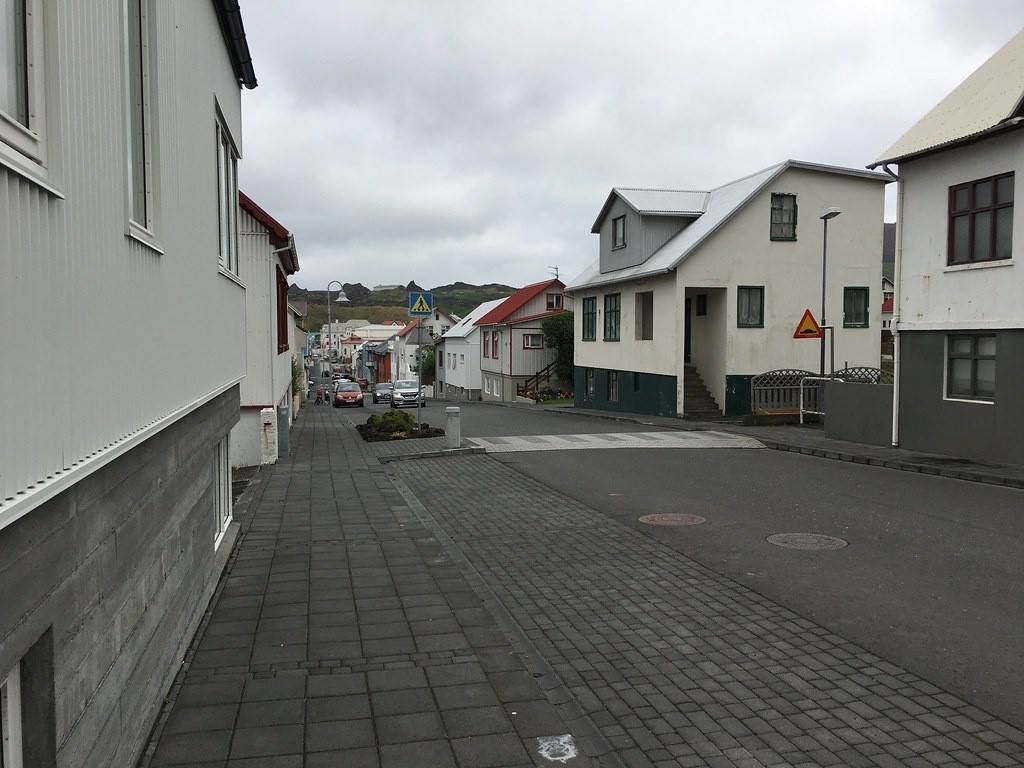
[389, 379, 426, 407]
[372, 381, 393, 403]
[306, 354, 370, 408]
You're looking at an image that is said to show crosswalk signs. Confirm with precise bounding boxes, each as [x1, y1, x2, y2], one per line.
[409, 292, 433, 316]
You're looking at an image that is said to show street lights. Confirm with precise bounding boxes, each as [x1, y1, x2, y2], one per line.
[820, 205, 845, 378]
[327, 281, 352, 410]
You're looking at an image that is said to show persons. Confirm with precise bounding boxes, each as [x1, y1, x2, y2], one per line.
[313, 389, 323, 405]
